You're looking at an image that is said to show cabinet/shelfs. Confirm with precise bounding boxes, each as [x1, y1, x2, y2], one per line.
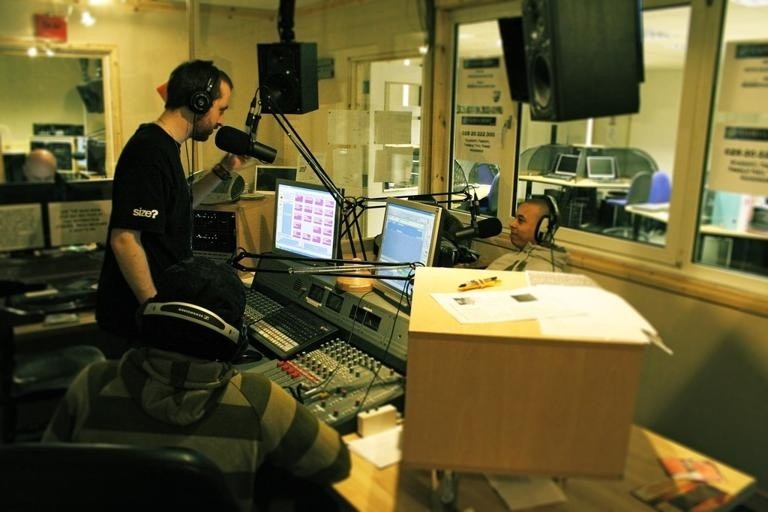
[398, 263, 653, 483]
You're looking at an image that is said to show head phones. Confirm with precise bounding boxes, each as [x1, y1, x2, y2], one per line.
[531, 195, 562, 248]
[138, 297, 250, 358]
[189, 65, 218, 115]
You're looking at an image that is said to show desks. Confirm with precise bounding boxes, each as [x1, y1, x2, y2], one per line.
[328, 398, 758, 512]
[622, 198, 768, 273]
[522, 168, 625, 191]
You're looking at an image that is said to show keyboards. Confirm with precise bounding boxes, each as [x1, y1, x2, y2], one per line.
[596, 180, 627, 185]
[543, 175, 572, 181]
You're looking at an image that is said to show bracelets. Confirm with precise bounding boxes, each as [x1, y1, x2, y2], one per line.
[211, 162, 231, 181]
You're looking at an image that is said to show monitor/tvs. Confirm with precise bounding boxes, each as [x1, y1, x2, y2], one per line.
[554, 153, 580, 177]
[86, 140, 106, 176]
[30, 141, 72, 171]
[3, 154, 28, 182]
[587, 155, 616, 178]
[253, 165, 297, 196]
[271, 178, 345, 267]
[371, 197, 443, 309]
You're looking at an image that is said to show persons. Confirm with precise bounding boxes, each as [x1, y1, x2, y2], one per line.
[23, 147, 59, 184]
[39, 253, 354, 510]
[94, 57, 233, 360]
[479, 196, 571, 271]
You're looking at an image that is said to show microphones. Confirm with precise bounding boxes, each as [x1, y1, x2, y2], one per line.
[215, 126, 277, 163]
[454, 218, 503, 239]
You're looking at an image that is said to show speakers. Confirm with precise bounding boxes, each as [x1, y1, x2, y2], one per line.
[523, 1, 640, 122]
[256, 40, 319, 114]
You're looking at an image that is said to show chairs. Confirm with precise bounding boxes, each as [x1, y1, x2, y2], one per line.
[451, 159, 503, 219]
[599, 170, 653, 242]
[647, 172, 673, 239]
